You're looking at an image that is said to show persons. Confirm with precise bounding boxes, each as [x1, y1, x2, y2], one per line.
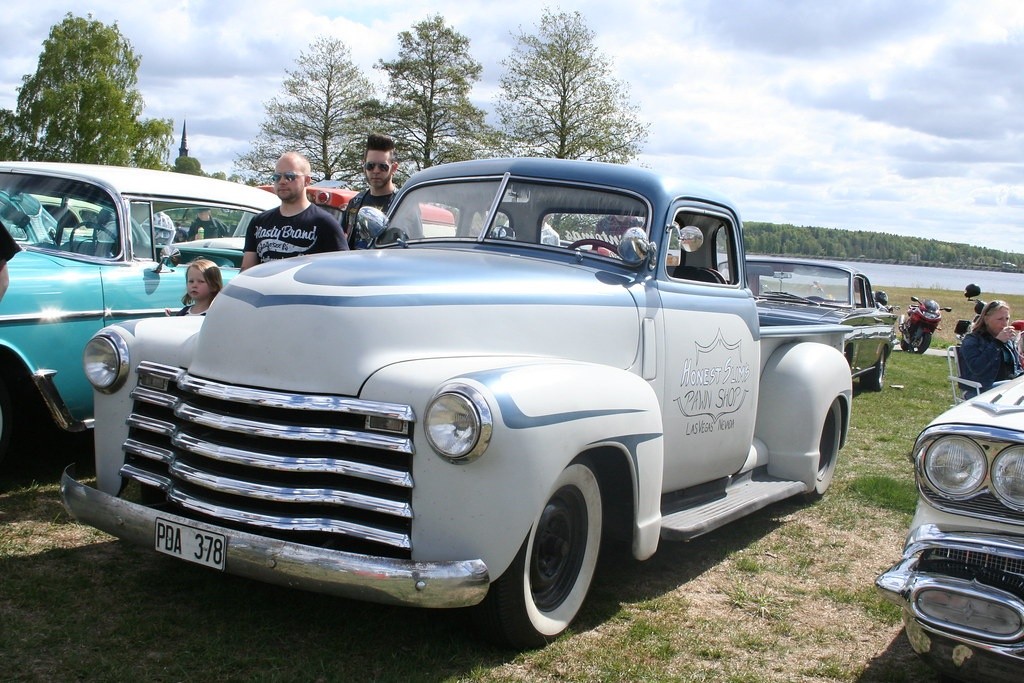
[592, 215, 640, 253]
[239, 150, 350, 273]
[501, 213, 561, 247]
[174, 208, 228, 242]
[164, 258, 223, 317]
[958, 300, 1024, 401]
[98, 208, 119, 255]
[342, 133, 424, 250]
[0, 220, 22, 302]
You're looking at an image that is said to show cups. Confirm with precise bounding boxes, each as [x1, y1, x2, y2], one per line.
[198, 227, 204, 239]
[1011, 330, 1020, 342]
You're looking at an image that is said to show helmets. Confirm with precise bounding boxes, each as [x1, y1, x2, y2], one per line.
[964, 284, 980, 297]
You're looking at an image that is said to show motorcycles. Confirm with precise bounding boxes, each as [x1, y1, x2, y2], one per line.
[953, 285, 1024, 374]
[897, 295, 952, 355]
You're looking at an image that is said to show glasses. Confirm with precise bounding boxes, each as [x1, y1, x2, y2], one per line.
[985, 301, 999, 314]
[365, 161, 395, 172]
[272, 171, 306, 181]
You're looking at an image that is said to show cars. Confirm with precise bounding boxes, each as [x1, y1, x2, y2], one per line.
[66, 159, 855, 655]
[0, 159, 278, 484]
[716, 255, 900, 392]
[875, 368, 1024, 683]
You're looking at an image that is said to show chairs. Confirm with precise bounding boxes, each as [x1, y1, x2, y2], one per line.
[947, 345, 983, 409]
[666, 265, 728, 286]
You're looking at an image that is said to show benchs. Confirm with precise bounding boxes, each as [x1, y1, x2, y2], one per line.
[61, 241, 245, 267]
